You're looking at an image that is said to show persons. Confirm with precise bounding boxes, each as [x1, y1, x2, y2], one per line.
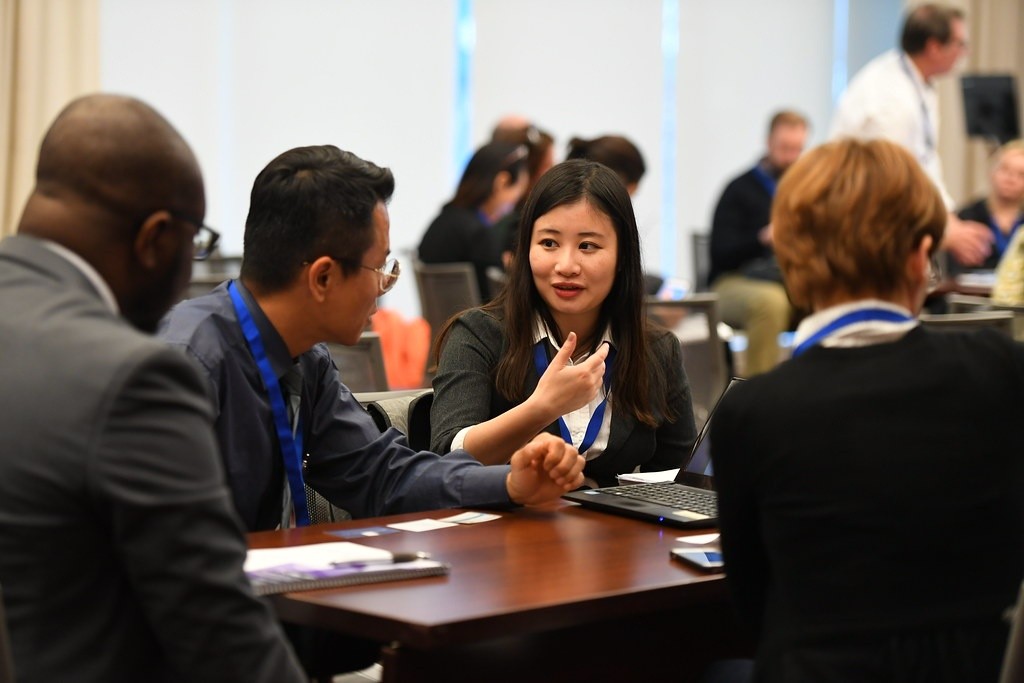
[707, 137, 1024, 683]
[705, 111, 809, 380]
[153, 144, 587, 534]
[0, 93, 304, 683]
[418, 117, 555, 307]
[430, 159, 698, 489]
[827, 5, 995, 295]
[947, 138, 1024, 280]
[491, 137, 646, 270]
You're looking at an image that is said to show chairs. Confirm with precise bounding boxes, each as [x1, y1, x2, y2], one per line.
[411, 252, 480, 384]
[693, 232, 711, 289]
[324, 331, 388, 393]
[920, 312, 1017, 340]
[644, 294, 726, 434]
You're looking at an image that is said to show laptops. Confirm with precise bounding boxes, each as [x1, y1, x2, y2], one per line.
[560, 375, 746, 527]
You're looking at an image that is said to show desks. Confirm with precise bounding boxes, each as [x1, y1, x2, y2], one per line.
[242, 495, 721, 653]
[352, 388, 436, 443]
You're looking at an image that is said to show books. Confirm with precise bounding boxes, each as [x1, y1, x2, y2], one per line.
[244, 542, 451, 595]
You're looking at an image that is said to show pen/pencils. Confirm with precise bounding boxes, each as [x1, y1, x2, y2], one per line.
[331, 551, 429, 569]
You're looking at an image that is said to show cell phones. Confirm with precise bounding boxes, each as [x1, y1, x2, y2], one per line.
[670, 547, 725, 574]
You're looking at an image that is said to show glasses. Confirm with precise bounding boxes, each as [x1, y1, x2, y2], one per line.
[926, 256, 941, 294]
[165, 209, 219, 261]
[332, 257, 401, 291]
